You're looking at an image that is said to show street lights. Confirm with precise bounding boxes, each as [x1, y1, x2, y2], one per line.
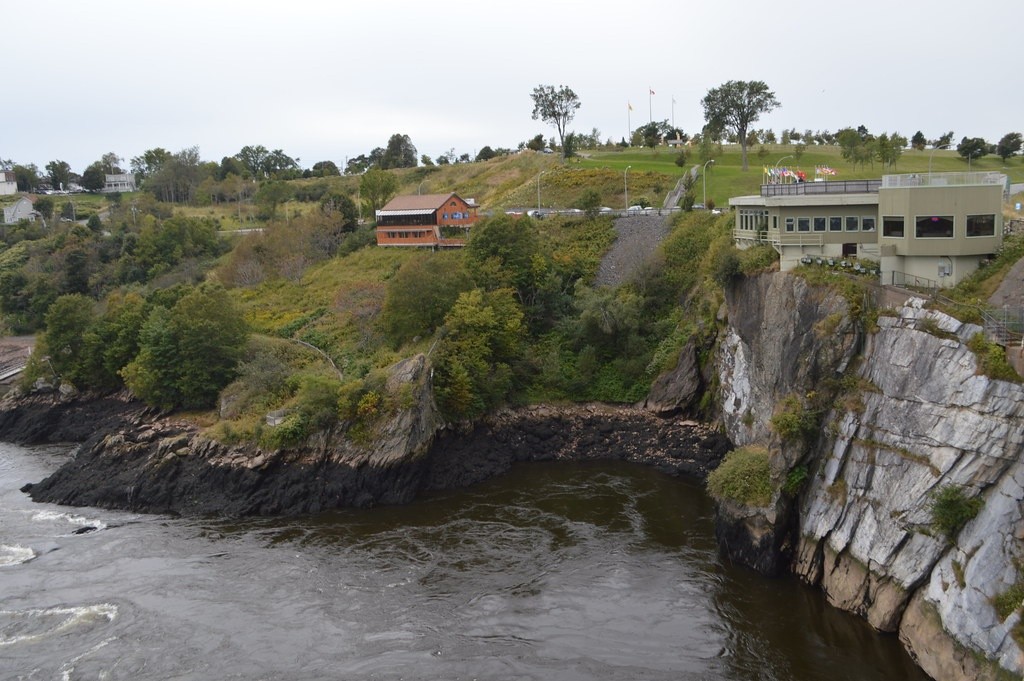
[418, 179, 432, 196]
[536, 171, 545, 215]
[928, 142, 957, 185]
[624, 165, 633, 212]
[703, 159, 714, 210]
[970, 148, 982, 174]
[775, 155, 793, 170]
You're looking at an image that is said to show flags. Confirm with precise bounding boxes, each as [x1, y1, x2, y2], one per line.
[764, 167, 807, 180]
[650, 89, 656, 95]
[815, 167, 839, 176]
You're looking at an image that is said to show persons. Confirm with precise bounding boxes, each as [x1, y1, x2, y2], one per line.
[799, 177, 803, 182]
